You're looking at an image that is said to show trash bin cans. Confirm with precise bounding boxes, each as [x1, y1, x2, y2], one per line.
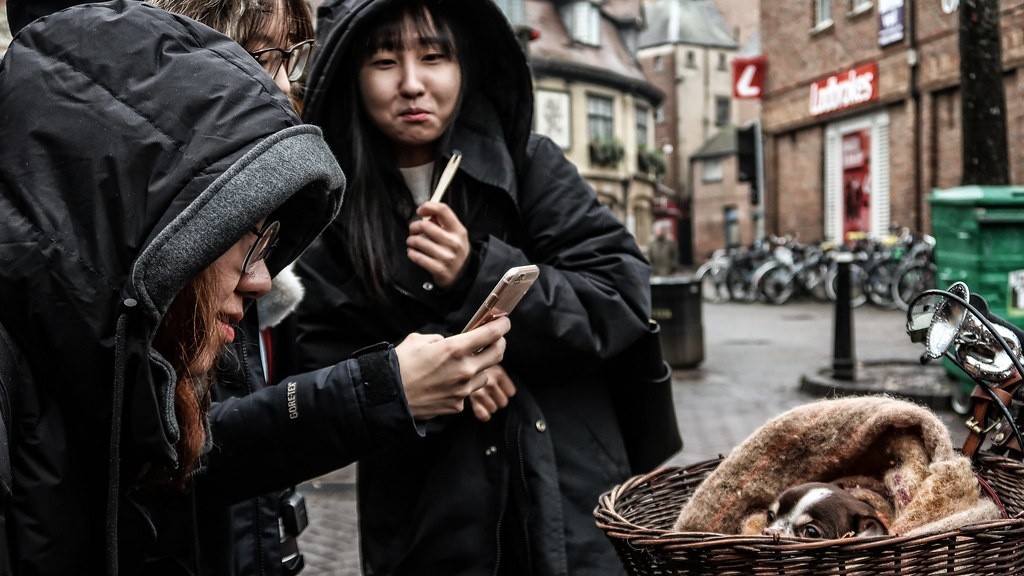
[652, 279, 705, 369]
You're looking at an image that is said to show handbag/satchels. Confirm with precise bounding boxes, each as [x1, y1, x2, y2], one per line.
[611, 318, 683, 476]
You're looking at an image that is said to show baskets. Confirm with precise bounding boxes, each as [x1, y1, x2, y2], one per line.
[593, 445, 1024, 576]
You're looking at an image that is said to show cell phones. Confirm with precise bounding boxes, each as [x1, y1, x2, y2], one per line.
[460, 263, 541, 359]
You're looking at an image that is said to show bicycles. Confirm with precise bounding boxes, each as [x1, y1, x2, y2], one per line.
[694, 220, 938, 313]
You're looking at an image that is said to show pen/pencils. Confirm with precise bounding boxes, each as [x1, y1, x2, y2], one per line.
[422, 150, 463, 220]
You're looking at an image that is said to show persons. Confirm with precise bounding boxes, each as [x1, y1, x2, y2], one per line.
[647, 221, 682, 276]
[300, 0, 655, 576]
[0, 3, 344, 576]
[142, 3, 517, 576]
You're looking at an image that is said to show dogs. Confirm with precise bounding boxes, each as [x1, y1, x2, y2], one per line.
[763, 481, 890, 541]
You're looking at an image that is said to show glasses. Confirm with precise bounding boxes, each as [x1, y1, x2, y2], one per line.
[242, 220, 282, 274]
[246, 38, 317, 81]
